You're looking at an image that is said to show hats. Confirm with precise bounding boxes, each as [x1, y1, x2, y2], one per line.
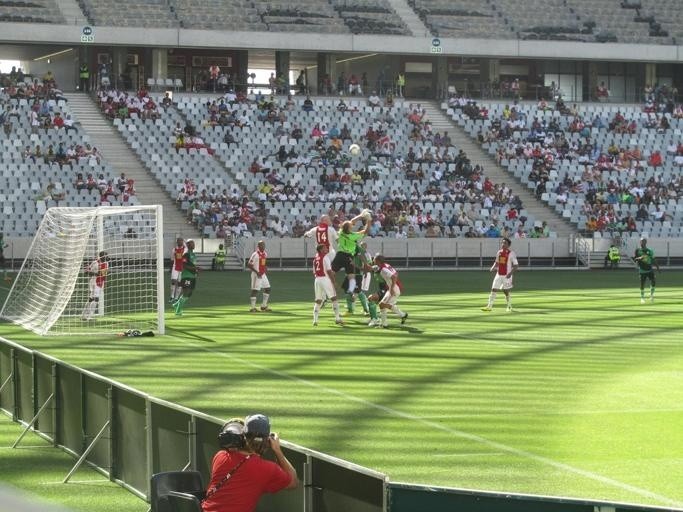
[246, 414, 269, 442]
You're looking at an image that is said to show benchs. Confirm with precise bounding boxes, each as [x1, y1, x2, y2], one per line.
[221, 81, 310, 93]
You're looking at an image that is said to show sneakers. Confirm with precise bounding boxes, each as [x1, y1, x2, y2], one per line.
[368, 317, 389, 328]
[336, 320, 345, 326]
[346, 290, 356, 303]
[641, 297, 647, 304]
[401, 313, 409, 324]
[314, 321, 317, 326]
[169, 297, 183, 316]
[82, 316, 97, 321]
[649, 295, 655, 306]
[251, 308, 258, 312]
[344, 310, 353, 315]
[481, 306, 493, 311]
[262, 306, 272, 311]
[364, 311, 372, 316]
[506, 305, 513, 313]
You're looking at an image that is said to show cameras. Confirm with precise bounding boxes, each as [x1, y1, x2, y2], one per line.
[217, 418, 274, 454]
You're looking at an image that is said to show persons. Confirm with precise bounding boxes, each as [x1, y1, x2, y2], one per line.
[313, 244, 343, 326]
[448, 78, 683, 239]
[359, 251, 408, 329]
[342, 241, 370, 317]
[320, 210, 372, 307]
[215, 244, 226, 271]
[225, 89, 265, 109]
[210, 64, 227, 91]
[291, 124, 302, 143]
[200, 416, 298, 512]
[123, 227, 138, 239]
[304, 95, 314, 114]
[269, 73, 287, 94]
[338, 98, 346, 115]
[296, 70, 305, 94]
[367, 256, 389, 327]
[304, 214, 339, 283]
[209, 99, 250, 147]
[364, 89, 451, 159]
[252, 145, 451, 238]
[256, 96, 295, 123]
[168, 238, 187, 303]
[0, 66, 136, 201]
[80, 251, 110, 322]
[480, 238, 518, 312]
[178, 178, 266, 239]
[172, 239, 200, 316]
[634, 238, 660, 304]
[175, 123, 203, 147]
[247, 240, 272, 312]
[139, 87, 171, 122]
[344, 241, 371, 313]
[605, 245, 621, 270]
[312, 124, 351, 149]
[324, 70, 405, 97]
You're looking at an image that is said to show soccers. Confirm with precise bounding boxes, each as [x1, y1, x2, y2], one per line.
[349, 144, 360, 154]
